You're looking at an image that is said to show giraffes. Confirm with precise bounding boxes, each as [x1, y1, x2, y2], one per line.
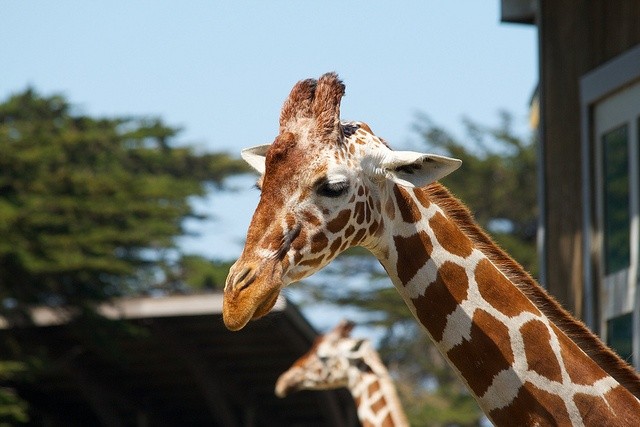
[274, 318, 408, 426]
[222, 71, 635, 426]
